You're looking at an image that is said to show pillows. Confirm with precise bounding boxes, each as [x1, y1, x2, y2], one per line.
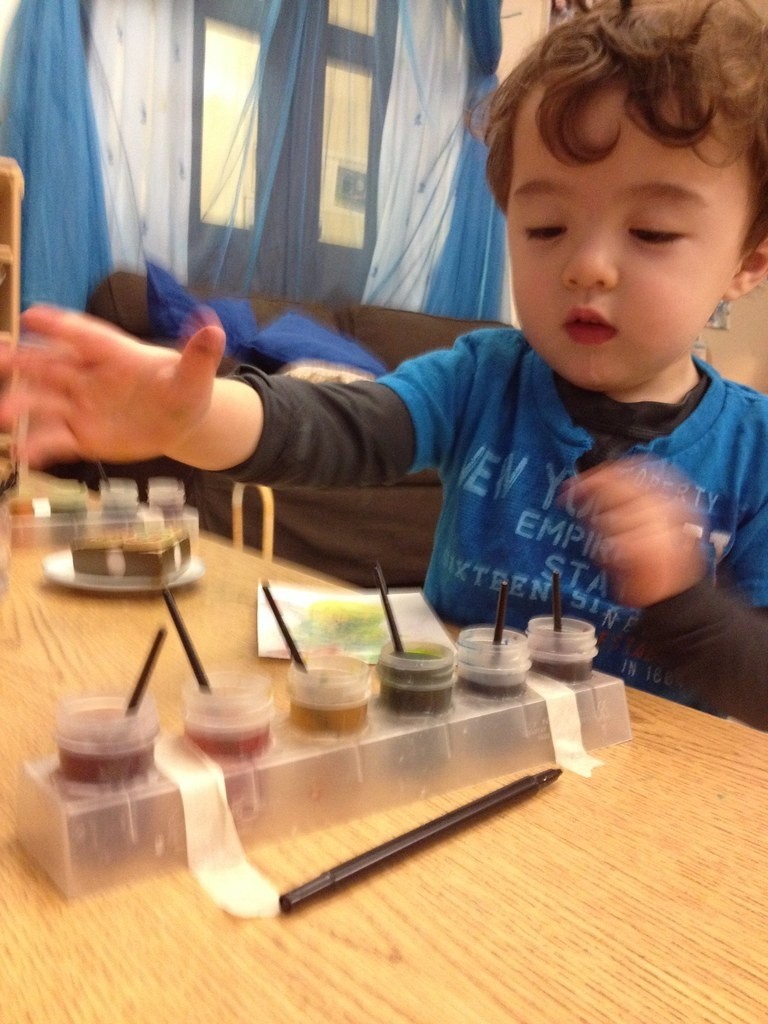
[248, 311, 386, 383]
[145, 259, 262, 376]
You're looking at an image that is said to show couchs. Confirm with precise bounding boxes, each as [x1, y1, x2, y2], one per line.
[80, 271, 518, 600]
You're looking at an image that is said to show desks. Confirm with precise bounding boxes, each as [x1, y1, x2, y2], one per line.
[0, 472, 767, 1024]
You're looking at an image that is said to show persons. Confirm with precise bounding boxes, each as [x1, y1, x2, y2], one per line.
[0, 0, 767, 731]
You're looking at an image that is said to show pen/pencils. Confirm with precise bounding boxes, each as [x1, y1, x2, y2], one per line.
[279, 766, 563, 913]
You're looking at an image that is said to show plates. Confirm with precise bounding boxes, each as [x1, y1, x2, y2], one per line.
[41, 549, 207, 594]
